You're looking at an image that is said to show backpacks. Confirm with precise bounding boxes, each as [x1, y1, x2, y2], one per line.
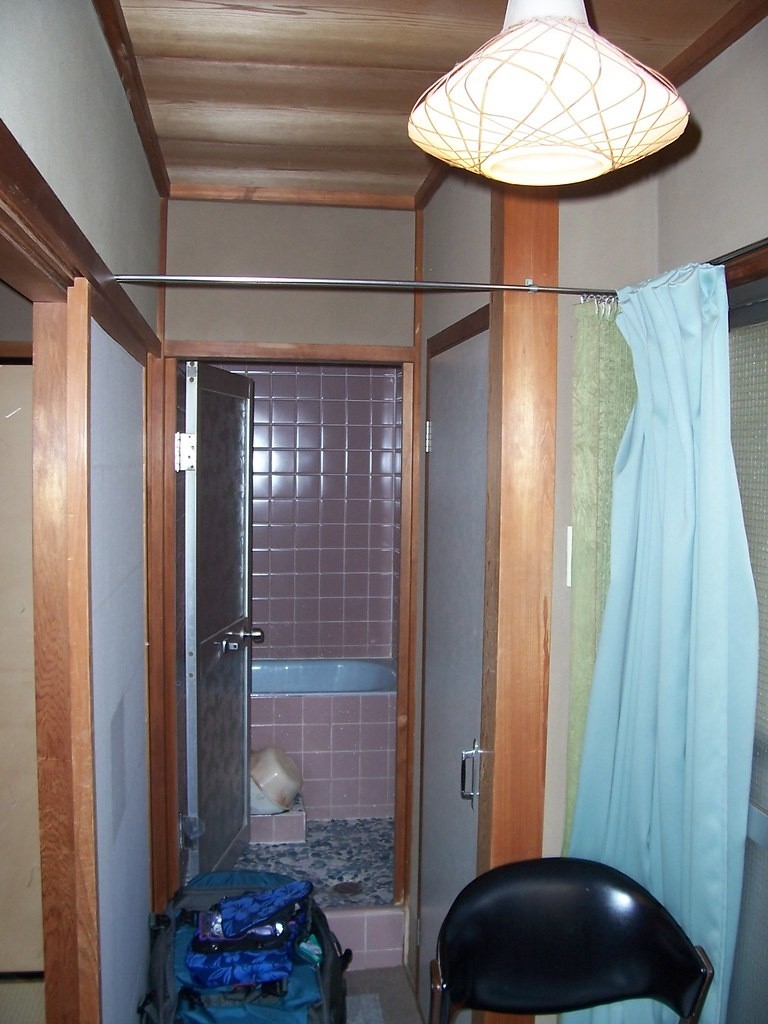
[178, 879, 315, 1006]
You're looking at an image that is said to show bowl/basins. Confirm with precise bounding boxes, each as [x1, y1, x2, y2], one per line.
[249, 746, 303, 811]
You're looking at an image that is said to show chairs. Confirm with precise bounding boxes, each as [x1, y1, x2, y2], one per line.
[429, 857, 714, 1024]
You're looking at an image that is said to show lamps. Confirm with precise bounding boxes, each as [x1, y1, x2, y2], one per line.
[407, 1, 690, 185]
